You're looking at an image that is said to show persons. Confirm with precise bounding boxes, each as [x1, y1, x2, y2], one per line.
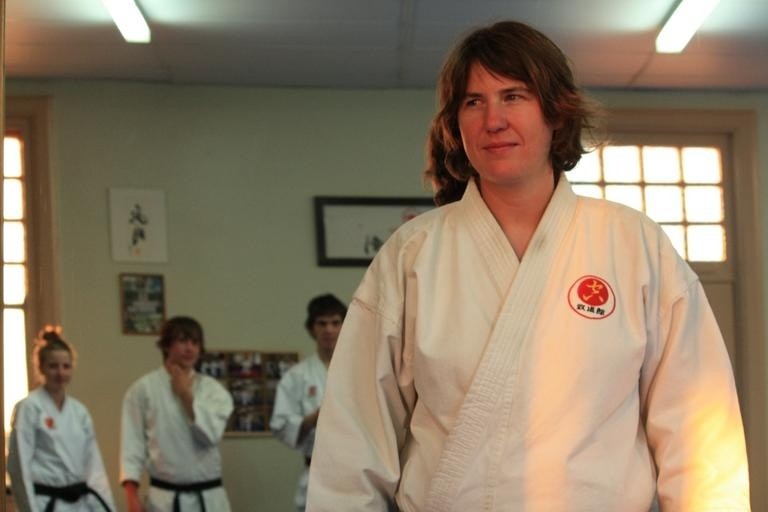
[266, 292, 347, 511]
[198, 350, 294, 431]
[117, 315, 235, 511]
[8, 325, 117, 511]
[126, 203, 149, 258]
[300, 17, 753, 512]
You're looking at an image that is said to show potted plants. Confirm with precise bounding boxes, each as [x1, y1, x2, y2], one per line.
[312, 195, 442, 268]
[195, 348, 305, 439]
[119, 272, 167, 337]
